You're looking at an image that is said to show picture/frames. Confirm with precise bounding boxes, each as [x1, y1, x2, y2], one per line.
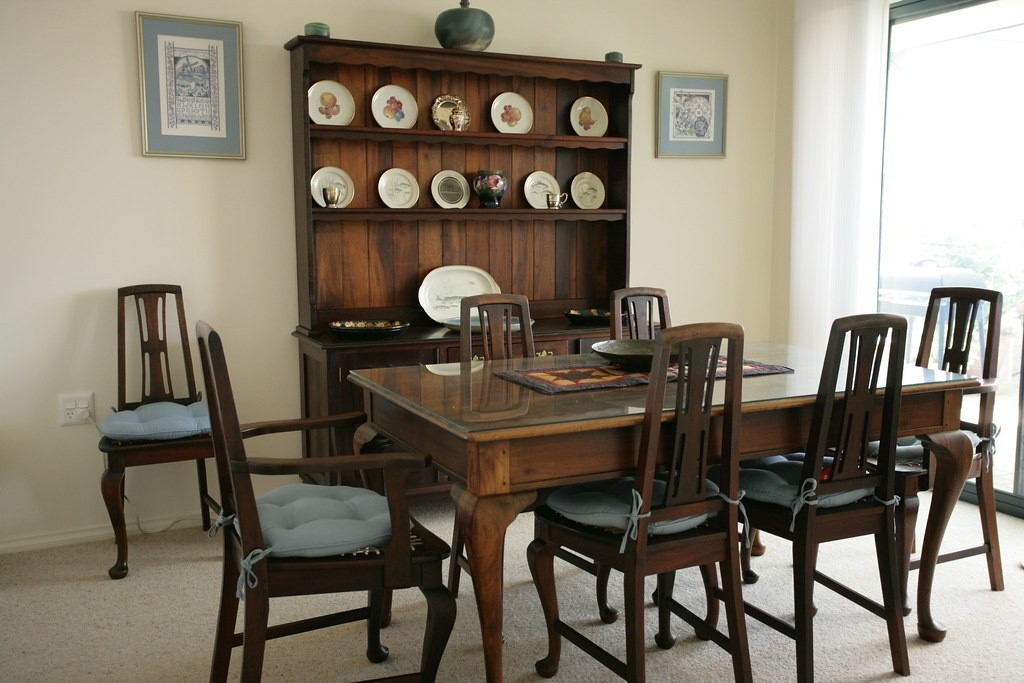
[135, 10, 246, 161]
[656, 71, 728, 158]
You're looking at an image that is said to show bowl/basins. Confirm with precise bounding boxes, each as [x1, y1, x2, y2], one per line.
[590, 339, 681, 373]
[563, 308, 626, 325]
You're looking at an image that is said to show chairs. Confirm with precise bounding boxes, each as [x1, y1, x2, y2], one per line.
[824, 287, 1003, 615]
[100, 284, 220, 578]
[527, 324, 753, 683]
[611, 288, 760, 601]
[460, 294, 620, 625]
[194, 319, 457, 683]
[694, 313, 911, 683]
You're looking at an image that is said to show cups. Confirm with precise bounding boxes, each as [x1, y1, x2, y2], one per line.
[604, 51, 623, 62]
[546, 192, 567, 209]
[322, 187, 339, 208]
[305, 23, 329, 40]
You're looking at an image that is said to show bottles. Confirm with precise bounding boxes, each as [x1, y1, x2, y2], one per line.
[432, 94, 472, 131]
[434, 0, 495, 52]
[449, 105, 464, 132]
[473, 169, 506, 208]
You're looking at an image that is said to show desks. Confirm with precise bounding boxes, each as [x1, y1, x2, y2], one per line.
[348, 340, 980, 683]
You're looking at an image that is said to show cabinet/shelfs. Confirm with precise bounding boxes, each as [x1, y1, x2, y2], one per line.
[283, 33, 643, 492]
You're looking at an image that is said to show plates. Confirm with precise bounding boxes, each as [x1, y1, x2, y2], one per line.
[371, 85, 418, 129]
[491, 92, 534, 134]
[311, 167, 354, 208]
[431, 169, 470, 209]
[524, 171, 560, 209]
[378, 167, 420, 208]
[418, 265, 500, 323]
[571, 171, 605, 209]
[308, 80, 356, 126]
[443, 315, 535, 333]
[329, 320, 411, 336]
[570, 97, 608, 136]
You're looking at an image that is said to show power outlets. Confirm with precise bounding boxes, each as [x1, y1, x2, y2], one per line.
[58, 392, 96, 426]
